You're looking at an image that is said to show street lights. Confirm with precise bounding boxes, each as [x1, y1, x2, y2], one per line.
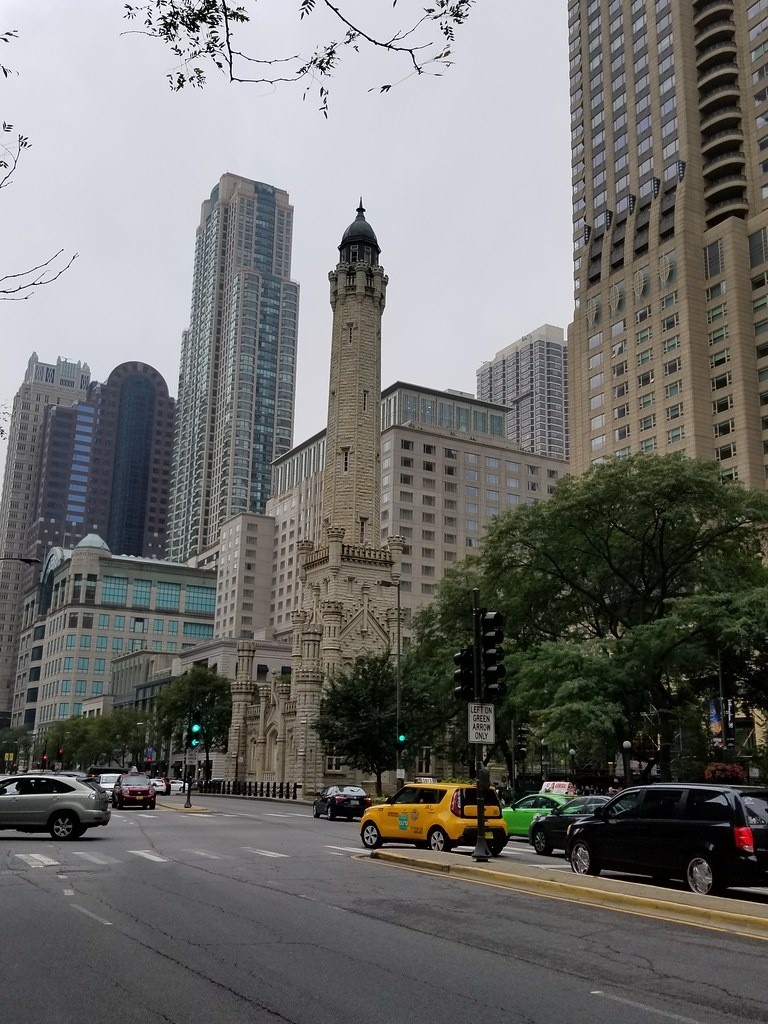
[377, 579, 403, 791]
[569, 749, 575, 783]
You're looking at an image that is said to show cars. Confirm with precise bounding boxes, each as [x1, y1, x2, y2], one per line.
[565, 783, 768, 895]
[112, 774, 156, 809]
[501, 794, 596, 837]
[313, 784, 372, 820]
[170, 780, 189, 791]
[360, 783, 509, 855]
[528, 796, 633, 856]
[149, 779, 166, 796]
[98, 774, 123, 801]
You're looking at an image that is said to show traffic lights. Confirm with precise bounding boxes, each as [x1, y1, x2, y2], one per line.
[148, 758, 151, 762]
[398, 731, 406, 747]
[453, 649, 474, 701]
[480, 608, 507, 701]
[189, 723, 201, 748]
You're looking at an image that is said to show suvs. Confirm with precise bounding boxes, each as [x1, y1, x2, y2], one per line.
[0, 771, 111, 841]
